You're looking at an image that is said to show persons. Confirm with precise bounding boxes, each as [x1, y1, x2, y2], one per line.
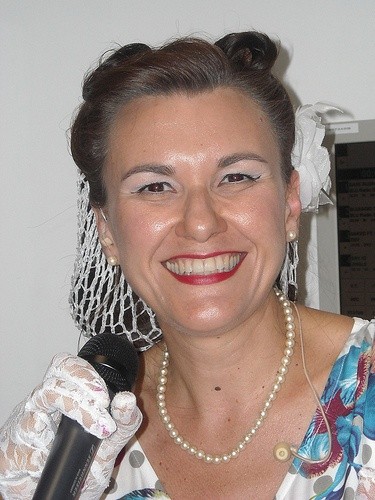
[0, 33, 374, 500]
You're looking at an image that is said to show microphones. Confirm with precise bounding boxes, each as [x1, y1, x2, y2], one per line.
[32, 333, 140, 500]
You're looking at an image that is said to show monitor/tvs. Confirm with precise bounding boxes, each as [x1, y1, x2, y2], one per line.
[315, 119, 375, 321]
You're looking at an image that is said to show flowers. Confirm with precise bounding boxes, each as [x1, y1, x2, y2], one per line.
[290, 102, 344, 215]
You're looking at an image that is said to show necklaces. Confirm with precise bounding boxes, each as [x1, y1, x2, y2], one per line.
[156, 285, 295, 464]
